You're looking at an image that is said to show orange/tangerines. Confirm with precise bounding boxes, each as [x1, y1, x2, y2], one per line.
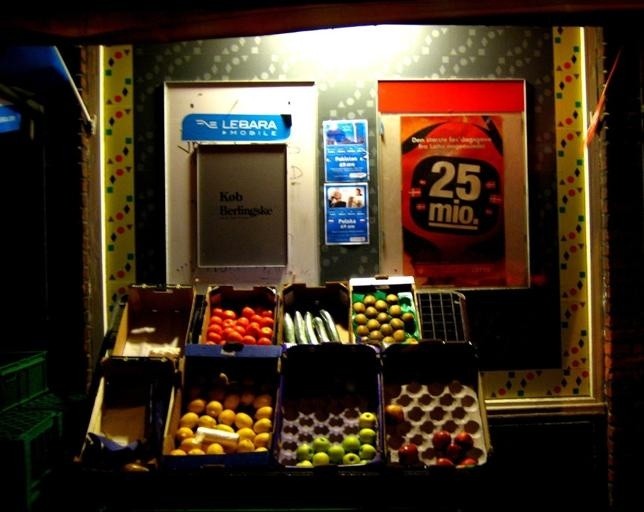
[352, 293, 419, 345]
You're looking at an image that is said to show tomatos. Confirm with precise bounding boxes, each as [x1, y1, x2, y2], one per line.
[206, 305, 274, 345]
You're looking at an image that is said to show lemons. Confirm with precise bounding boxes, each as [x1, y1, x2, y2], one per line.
[170, 387, 273, 456]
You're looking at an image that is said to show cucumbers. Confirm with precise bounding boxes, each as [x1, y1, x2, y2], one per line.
[283, 309, 341, 344]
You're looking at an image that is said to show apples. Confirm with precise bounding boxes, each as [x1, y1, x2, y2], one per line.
[296, 412, 377, 468]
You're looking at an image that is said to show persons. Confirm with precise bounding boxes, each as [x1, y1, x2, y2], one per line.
[349, 188, 363, 208]
[330, 190, 342, 207]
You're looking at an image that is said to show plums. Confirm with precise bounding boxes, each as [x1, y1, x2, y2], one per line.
[433, 431, 477, 465]
[386, 405, 404, 424]
[398, 444, 418, 462]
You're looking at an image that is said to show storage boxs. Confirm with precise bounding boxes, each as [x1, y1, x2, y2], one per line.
[161, 356, 283, 479]
[278, 281, 349, 345]
[73, 358, 177, 471]
[349, 276, 422, 344]
[381, 340, 494, 477]
[185, 284, 283, 357]
[270, 342, 384, 477]
[111, 284, 196, 358]
